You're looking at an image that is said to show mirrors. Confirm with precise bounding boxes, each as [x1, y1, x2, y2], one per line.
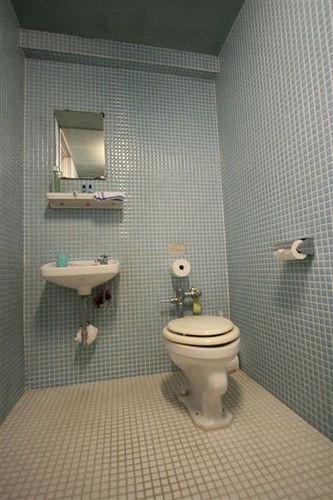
[53, 109, 107, 180]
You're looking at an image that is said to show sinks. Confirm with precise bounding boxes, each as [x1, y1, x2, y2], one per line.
[40, 260, 120, 296]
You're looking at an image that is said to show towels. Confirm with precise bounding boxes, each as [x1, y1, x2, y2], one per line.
[94, 191, 126, 203]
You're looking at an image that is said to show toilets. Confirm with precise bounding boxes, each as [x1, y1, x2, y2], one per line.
[163, 315, 241, 430]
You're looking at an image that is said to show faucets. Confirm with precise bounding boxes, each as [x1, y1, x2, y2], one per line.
[95, 254, 109, 265]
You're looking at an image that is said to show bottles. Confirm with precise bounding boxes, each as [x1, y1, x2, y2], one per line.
[51, 170, 62, 192]
